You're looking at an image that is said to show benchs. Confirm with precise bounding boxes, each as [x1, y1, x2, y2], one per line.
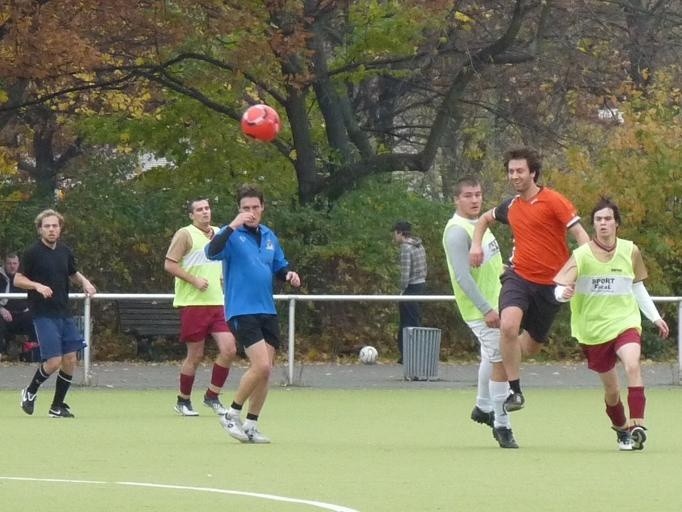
[116, 299, 179, 358]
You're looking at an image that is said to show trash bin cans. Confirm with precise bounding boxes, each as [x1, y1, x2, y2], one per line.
[402, 326, 442, 382]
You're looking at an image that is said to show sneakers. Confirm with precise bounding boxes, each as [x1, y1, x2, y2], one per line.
[21, 386, 36, 415]
[202, 392, 229, 415]
[174, 395, 200, 417]
[47, 404, 75, 420]
[471, 391, 527, 449]
[219, 411, 272, 443]
[610, 423, 647, 452]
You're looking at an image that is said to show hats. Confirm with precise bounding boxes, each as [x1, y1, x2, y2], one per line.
[390, 222, 412, 233]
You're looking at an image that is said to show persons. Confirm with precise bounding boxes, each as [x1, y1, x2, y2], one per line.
[203, 185, 301, 444]
[12, 208, 97, 417]
[391, 222, 427, 365]
[0, 254, 42, 362]
[468, 145, 589, 412]
[163, 199, 237, 416]
[552, 199, 669, 451]
[442, 177, 519, 448]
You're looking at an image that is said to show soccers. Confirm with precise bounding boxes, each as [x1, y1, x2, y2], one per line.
[361, 346, 380, 365]
[241, 104, 280, 143]
